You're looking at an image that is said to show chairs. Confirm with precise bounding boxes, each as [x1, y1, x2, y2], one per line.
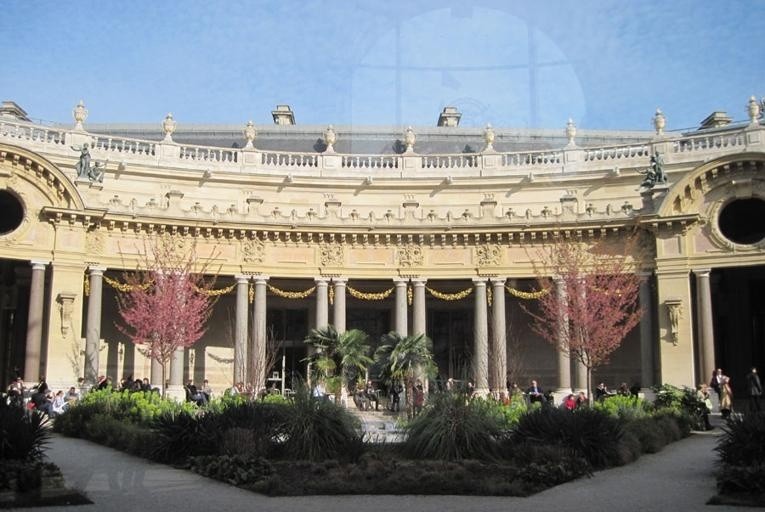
[184, 376, 385, 411]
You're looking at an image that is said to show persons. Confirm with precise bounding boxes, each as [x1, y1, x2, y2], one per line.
[564, 380, 641, 411]
[310, 377, 554, 417]
[696, 368, 734, 430]
[70, 142, 91, 177]
[89, 159, 106, 181]
[635, 150, 669, 192]
[183, 378, 276, 409]
[744, 366, 763, 412]
[0, 374, 162, 420]
